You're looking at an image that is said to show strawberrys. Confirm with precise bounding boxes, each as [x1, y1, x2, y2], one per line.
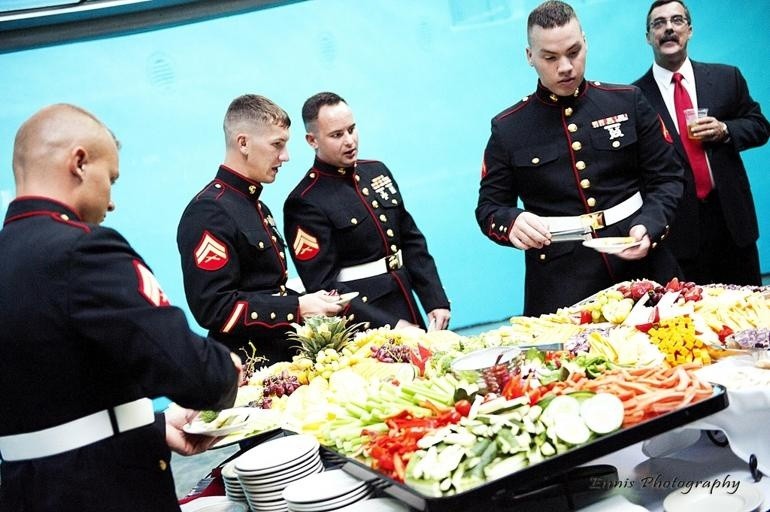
[664, 277, 703, 303]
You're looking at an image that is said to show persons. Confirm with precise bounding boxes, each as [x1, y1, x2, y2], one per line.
[631, 2, 770, 286]
[282, 96, 453, 336]
[468, 2, 691, 317]
[1, 103, 243, 510]
[176, 93, 350, 375]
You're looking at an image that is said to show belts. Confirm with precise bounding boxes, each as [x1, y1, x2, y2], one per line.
[0, 394, 157, 466]
[334, 247, 406, 285]
[538, 187, 644, 244]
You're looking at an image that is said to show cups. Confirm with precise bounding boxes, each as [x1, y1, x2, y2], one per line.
[682, 107, 709, 140]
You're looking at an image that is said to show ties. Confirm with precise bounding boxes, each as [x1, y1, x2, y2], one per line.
[671, 70, 714, 202]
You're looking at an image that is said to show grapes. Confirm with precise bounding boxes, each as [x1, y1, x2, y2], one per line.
[579, 290, 623, 323]
[370, 339, 411, 364]
[255, 369, 300, 409]
[311, 345, 351, 379]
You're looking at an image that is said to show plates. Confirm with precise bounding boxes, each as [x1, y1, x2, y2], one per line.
[332, 292, 359, 305]
[582, 237, 644, 256]
[663, 477, 764, 512]
[181, 407, 262, 439]
[179, 494, 249, 512]
[220, 434, 374, 512]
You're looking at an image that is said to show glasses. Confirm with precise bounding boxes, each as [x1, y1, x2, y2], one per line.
[649, 14, 687, 30]
